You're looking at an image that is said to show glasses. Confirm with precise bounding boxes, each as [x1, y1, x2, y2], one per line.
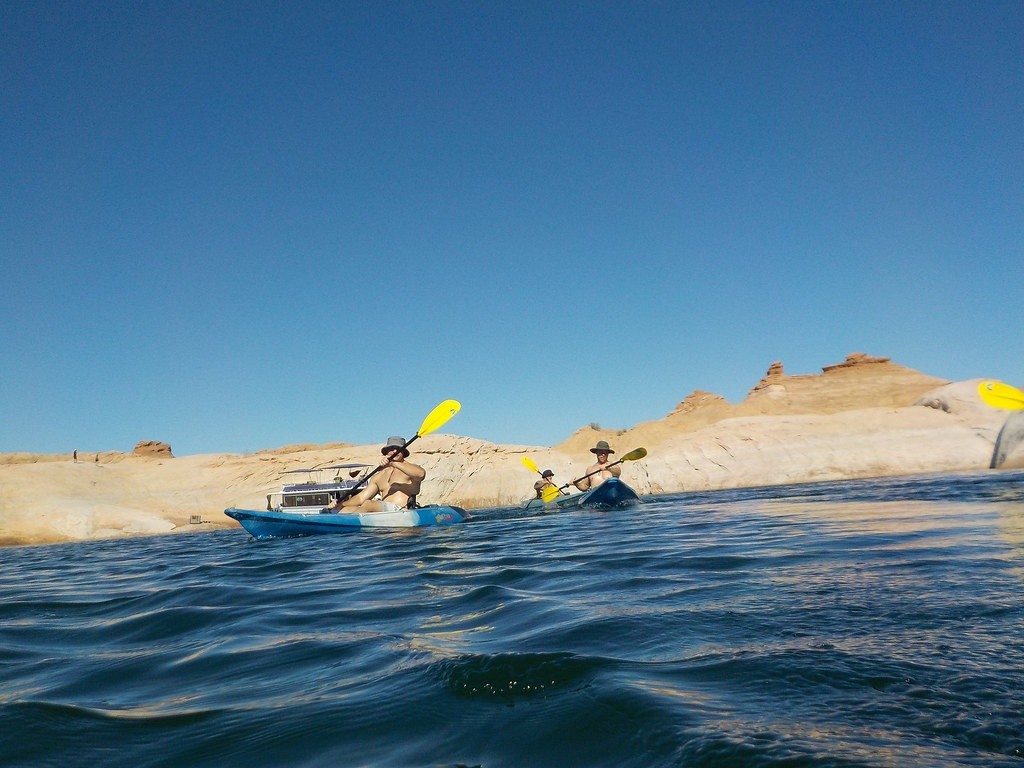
[389, 448, 400, 451]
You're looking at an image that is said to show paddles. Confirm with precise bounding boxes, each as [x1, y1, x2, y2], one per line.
[319, 398, 463, 514]
[522, 447, 647, 503]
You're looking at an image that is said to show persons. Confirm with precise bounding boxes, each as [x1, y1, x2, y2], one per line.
[534, 470, 563, 499]
[330, 436, 426, 513]
[95, 454, 100, 466]
[74, 449, 78, 463]
[569, 441, 622, 492]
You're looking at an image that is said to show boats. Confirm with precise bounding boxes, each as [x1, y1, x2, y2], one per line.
[519, 475, 641, 510]
[224, 504, 472, 541]
[267, 463, 376, 515]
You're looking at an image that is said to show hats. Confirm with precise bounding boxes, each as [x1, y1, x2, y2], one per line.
[590, 441, 615, 453]
[381, 436, 410, 458]
[542, 470, 554, 478]
